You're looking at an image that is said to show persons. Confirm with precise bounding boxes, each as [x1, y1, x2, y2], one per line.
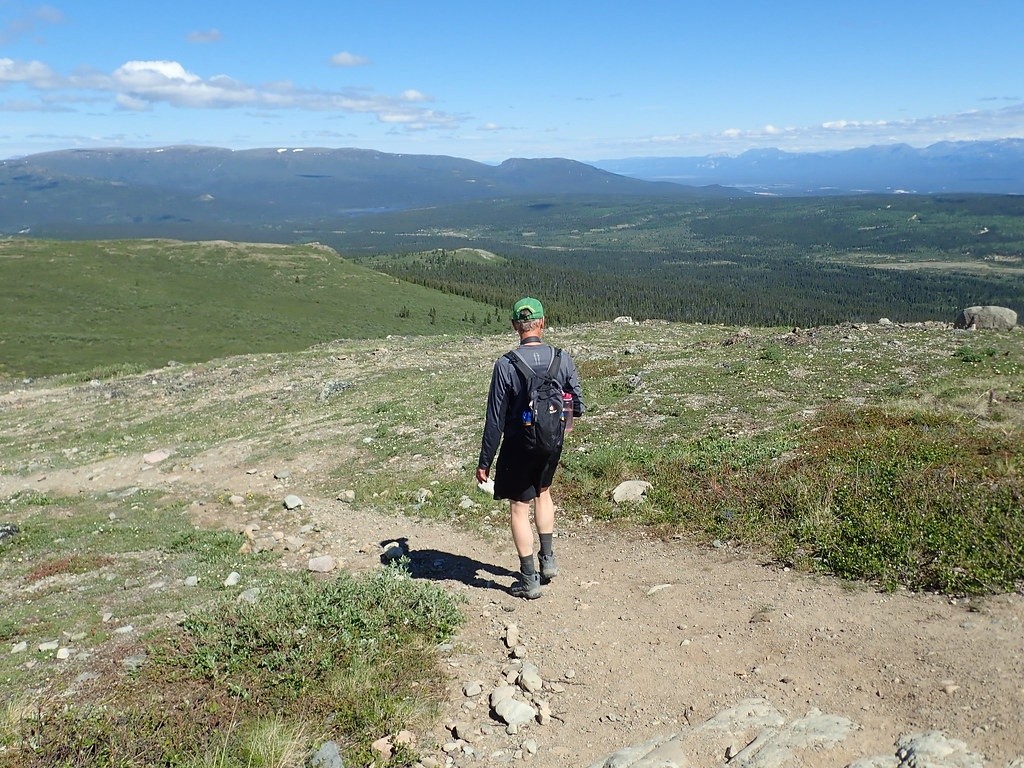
[477, 297, 586, 600]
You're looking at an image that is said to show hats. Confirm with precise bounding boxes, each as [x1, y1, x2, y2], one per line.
[513, 297, 543, 319]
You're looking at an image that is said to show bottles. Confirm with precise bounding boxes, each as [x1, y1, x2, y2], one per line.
[563, 393, 573, 433]
[520, 411, 532, 428]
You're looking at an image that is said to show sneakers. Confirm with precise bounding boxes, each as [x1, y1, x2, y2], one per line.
[537, 550, 558, 578]
[510, 571, 542, 598]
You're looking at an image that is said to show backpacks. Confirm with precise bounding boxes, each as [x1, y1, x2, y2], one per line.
[504, 346, 565, 457]
